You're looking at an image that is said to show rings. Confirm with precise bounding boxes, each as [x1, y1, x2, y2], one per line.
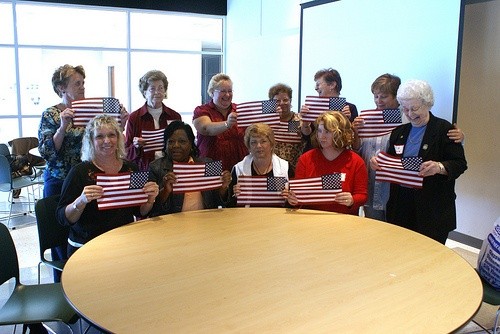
[346, 200, 348, 203]
[164, 176, 168, 180]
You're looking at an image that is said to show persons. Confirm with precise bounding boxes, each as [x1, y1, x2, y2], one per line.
[192, 73, 249, 171]
[266, 83, 308, 170]
[54, 113, 159, 257]
[352, 73, 465, 221]
[124, 70, 182, 221]
[288, 110, 369, 216]
[299, 68, 358, 152]
[38, 64, 130, 283]
[226, 122, 295, 208]
[369, 80, 468, 245]
[146, 120, 232, 218]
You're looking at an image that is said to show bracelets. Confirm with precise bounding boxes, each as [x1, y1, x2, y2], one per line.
[438, 161, 446, 175]
[72, 201, 82, 212]
[148, 197, 156, 204]
[224, 121, 230, 129]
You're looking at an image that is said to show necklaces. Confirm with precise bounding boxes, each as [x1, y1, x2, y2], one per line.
[280, 111, 293, 119]
[253, 160, 271, 176]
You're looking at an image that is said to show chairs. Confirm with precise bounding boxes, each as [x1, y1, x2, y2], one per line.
[0, 137, 45, 230]
[35, 194, 83, 334]
[0, 223, 91, 334]
[451, 224, 500, 334]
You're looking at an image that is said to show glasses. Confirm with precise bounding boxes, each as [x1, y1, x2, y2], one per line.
[316, 82, 327, 86]
[401, 103, 423, 112]
[214, 89, 233, 94]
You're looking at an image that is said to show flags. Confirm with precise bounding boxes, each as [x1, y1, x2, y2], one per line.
[271, 121, 303, 142]
[236, 99, 281, 127]
[236, 176, 288, 204]
[172, 159, 225, 192]
[300, 96, 346, 122]
[142, 128, 165, 152]
[357, 109, 404, 138]
[97, 170, 156, 210]
[288, 173, 342, 203]
[375, 151, 423, 189]
[71, 97, 124, 126]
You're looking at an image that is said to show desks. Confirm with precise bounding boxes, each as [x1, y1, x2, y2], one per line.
[61, 207, 484, 334]
